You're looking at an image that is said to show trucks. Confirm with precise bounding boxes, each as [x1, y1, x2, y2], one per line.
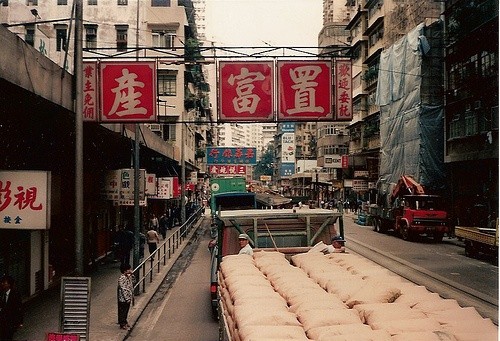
[217, 207, 496, 340]
[210, 191, 257, 211]
[455, 217, 499, 256]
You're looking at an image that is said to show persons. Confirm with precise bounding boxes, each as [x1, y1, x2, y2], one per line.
[237, 234, 253, 257]
[330, 236, 346, 255]
[118, 263, 135, 332]
[109, 199, 204, 264]
[248, 182, 378, 215]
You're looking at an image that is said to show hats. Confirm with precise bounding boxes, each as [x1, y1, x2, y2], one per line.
[332, 236, 346, 242]
[238, 233, 249, 240]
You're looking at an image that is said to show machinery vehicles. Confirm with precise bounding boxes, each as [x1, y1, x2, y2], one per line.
[367, 173, 450, 244]
